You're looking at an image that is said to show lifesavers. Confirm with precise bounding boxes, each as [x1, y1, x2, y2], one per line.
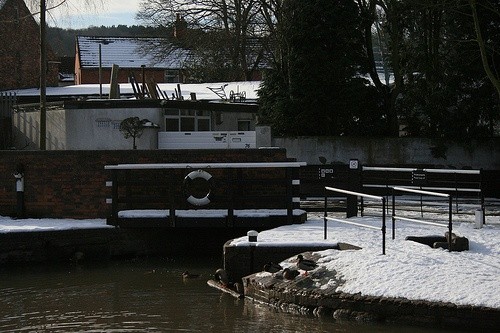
[182, 170, 216, 206]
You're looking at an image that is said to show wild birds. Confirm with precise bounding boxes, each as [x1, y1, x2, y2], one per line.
[215, 268, 230, 288]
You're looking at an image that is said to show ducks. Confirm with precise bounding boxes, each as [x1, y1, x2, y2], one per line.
[296, 254, 319, 276]
[183, 271, 200, 279]
[264, 264, 283, 275]
[282, 268, 300, 280]
